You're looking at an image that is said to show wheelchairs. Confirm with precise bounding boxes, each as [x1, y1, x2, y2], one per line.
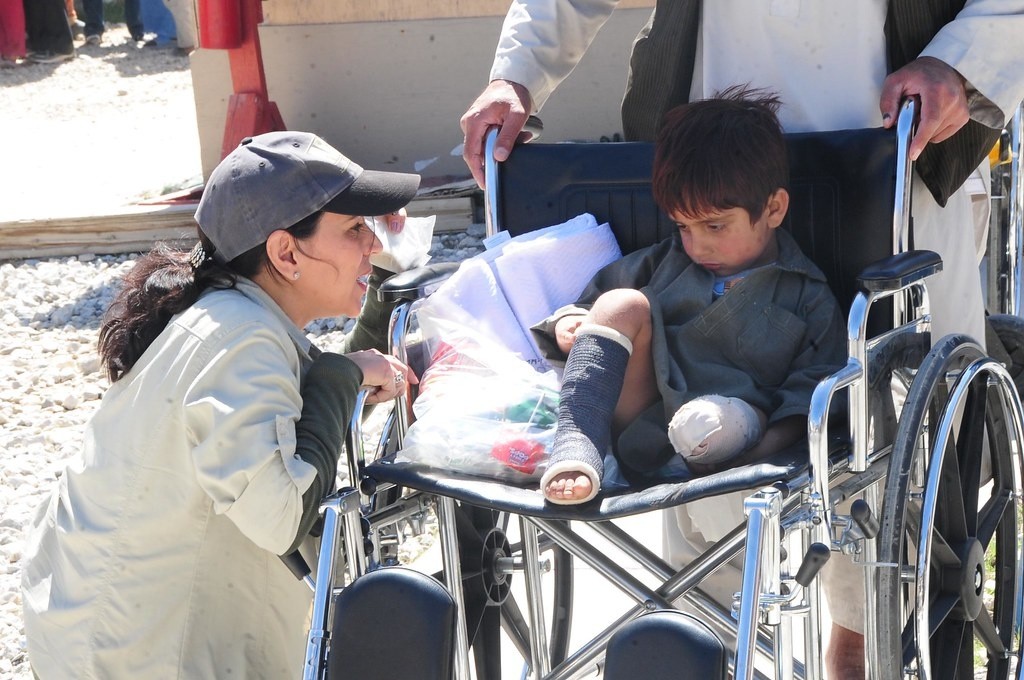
[285, 93, 1024, 678]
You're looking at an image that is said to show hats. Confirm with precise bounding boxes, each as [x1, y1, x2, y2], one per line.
[194, 131, 420, 265]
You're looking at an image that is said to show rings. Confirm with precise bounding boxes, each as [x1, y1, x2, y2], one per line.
[394, 370, 404, 384]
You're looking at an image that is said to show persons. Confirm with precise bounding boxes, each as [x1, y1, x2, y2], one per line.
[23, 0, 76, 63]
[22, 132, 421, 680]
[81, 0, 144, 45]
[457, 0, 1022, 680]
[530, 82, 847, 504]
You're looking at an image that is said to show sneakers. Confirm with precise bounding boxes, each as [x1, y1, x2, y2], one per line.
[28, 50, 76, 64]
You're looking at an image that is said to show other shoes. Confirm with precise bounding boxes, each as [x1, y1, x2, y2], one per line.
[170, 46, 193, 55]
[86, 34, 100, 43]
